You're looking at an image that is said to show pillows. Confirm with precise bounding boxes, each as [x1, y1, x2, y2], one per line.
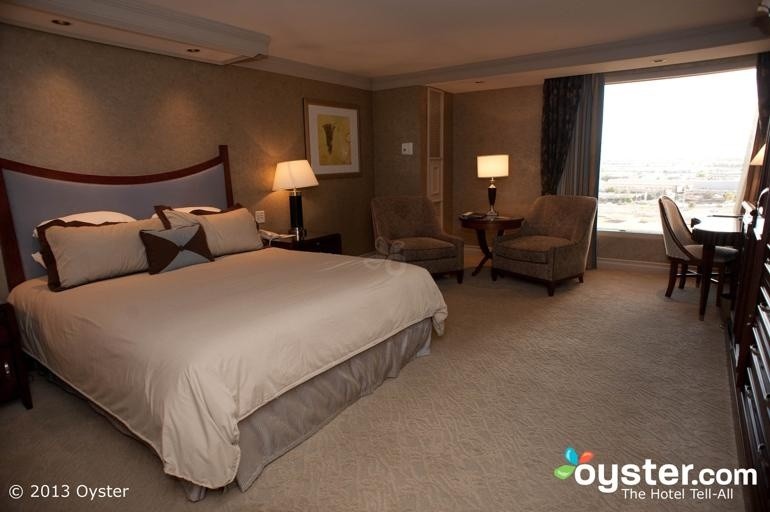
[153, 203, 262, 258]
[32, 210, 137, 239]
[35, 218, 164, 292]
[138, 222, 214, 275]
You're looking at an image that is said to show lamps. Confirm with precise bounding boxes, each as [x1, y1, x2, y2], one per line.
[476, 154, 509, 215]
[272, 160, 319, 241]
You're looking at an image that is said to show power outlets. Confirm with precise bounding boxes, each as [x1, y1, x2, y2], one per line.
[402, 142, 413, 155]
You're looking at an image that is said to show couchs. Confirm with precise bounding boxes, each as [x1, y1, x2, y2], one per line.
[491, 197, 597, 296]
[370, 196, 464, 284]
[658, 196, 738, 297]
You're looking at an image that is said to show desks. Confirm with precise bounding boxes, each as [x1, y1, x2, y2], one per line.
[691, 214, 742, 320]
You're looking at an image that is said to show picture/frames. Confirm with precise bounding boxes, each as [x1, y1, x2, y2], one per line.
[303, 97, 362, 180]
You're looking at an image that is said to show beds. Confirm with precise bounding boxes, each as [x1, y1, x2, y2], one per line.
[0, 143, 447, 502]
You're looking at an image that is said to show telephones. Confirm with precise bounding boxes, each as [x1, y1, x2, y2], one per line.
[258, 228, 280, 244]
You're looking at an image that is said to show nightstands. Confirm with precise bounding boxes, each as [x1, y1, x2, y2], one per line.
[0, 303, 32, 410]
[263, 232, 341, 253]
